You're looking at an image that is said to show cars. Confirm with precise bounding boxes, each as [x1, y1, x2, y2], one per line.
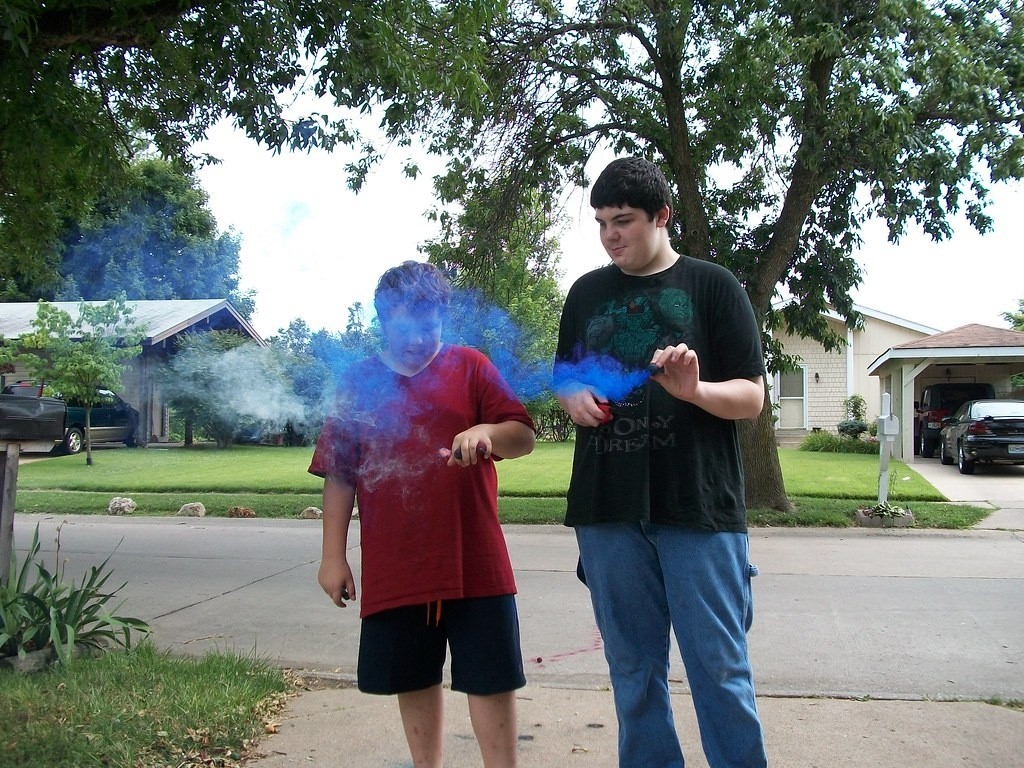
[938, 398, 1024, 475]
[0, 378, 141, 455]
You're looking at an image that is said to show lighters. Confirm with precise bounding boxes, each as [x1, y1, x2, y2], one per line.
[596, 404, 614, 421]
[342, 589, 350, 602]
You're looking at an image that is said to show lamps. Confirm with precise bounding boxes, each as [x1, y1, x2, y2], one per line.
[815, 373, 819, 382]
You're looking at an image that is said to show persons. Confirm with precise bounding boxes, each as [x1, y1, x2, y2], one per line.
[307, 260, 536, 768]
[553, 156, 766, 768]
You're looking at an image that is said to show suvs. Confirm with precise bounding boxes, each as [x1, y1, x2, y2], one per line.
[914, 382, 996, 457]
